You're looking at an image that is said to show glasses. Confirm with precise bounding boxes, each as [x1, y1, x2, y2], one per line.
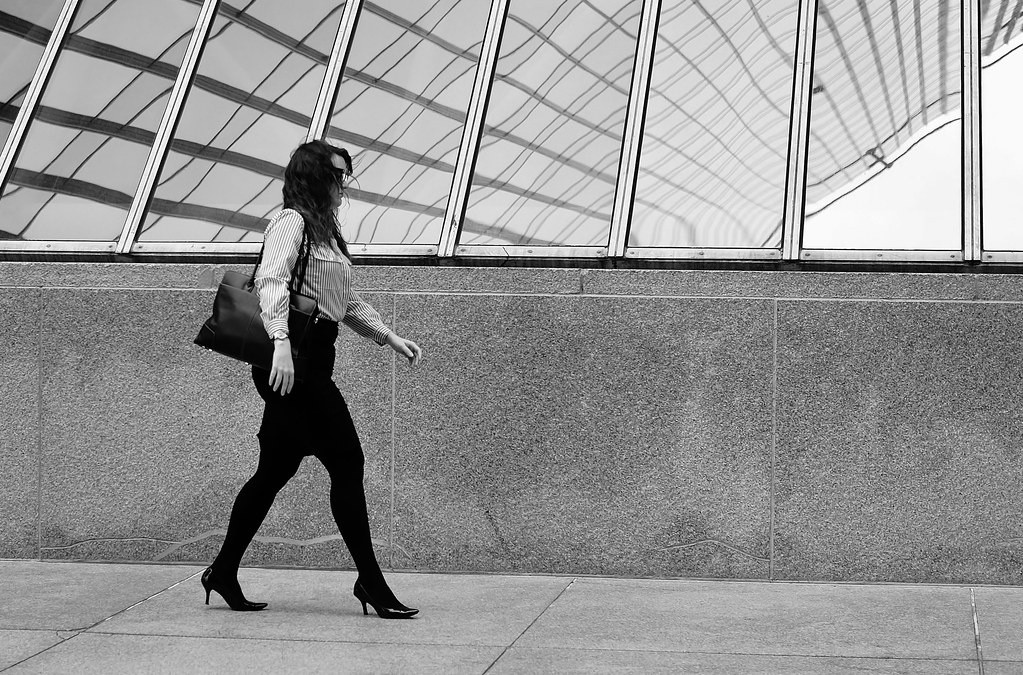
[323, 167, 349, 184]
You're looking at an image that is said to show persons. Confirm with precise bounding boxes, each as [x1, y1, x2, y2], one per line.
[201, 140, 422, 619]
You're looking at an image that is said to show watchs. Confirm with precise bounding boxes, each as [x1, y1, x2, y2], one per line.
[269, 330, 290, 344]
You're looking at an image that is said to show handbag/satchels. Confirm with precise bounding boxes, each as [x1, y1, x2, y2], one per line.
[193, 207, 321, 382]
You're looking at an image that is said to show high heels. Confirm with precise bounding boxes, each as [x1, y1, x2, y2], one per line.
[201, 567, 267, 611]
[353, 579, 419, 619]
